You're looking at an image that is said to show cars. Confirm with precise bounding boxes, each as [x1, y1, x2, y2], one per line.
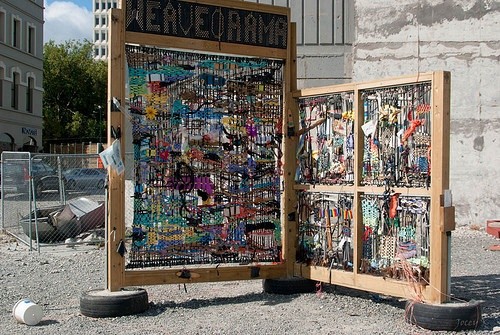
[62, 168, 107, 191]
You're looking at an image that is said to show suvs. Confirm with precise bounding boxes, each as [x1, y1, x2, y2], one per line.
[1, 158, 67, 199]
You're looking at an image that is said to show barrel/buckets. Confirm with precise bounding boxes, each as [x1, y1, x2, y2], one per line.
[12, 298, 43, 326]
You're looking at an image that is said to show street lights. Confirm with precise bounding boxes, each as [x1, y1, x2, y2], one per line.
[98, 106, 103, 144]
